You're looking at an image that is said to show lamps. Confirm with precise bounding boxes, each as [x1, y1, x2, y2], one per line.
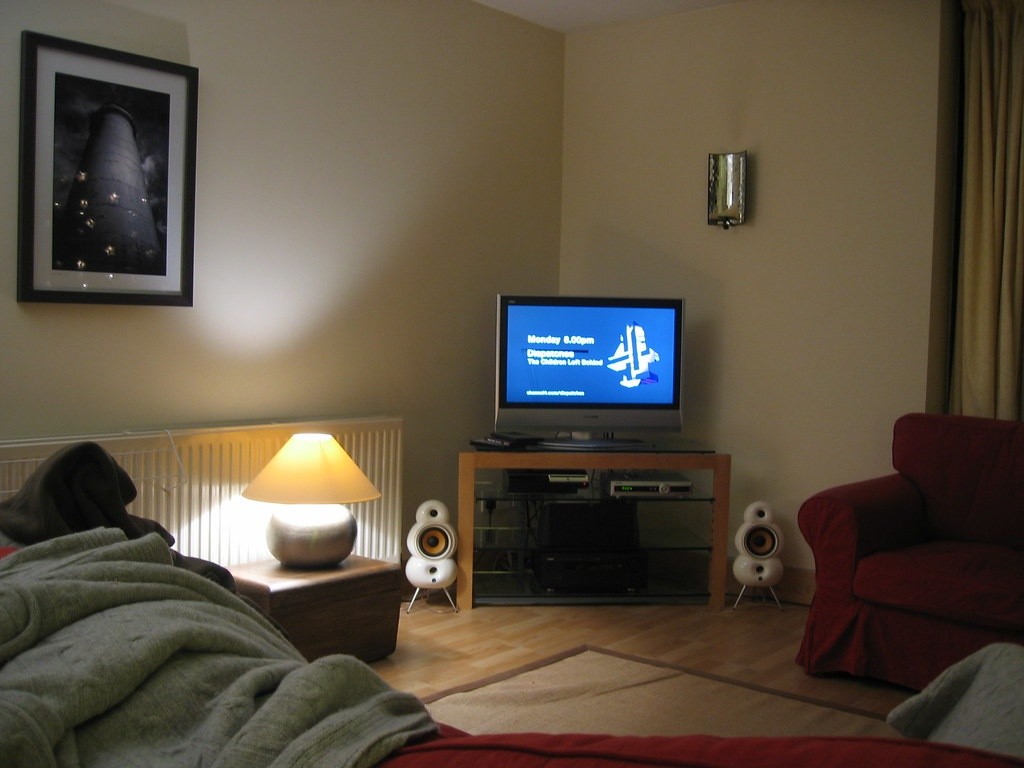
[707, 150, 747, 230]
[241, 433, 382, 571]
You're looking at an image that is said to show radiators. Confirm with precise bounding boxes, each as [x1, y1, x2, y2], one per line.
[0, 415, 405, 568]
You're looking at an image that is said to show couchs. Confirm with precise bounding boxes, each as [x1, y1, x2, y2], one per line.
[794, 412, 1024, 691]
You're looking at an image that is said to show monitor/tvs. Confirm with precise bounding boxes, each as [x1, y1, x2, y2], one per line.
[494, 294, 687, 450]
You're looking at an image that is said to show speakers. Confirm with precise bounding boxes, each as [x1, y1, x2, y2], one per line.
[405, 500, 458, 590]
[732, 500, 783, 588]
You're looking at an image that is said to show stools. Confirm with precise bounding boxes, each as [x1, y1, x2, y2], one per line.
[222, 554, 402, 665]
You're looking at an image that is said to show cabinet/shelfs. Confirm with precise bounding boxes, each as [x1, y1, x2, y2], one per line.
[455, 443, 731, 613]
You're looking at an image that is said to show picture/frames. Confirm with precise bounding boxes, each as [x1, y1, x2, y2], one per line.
[18, 30, 199, 308]
